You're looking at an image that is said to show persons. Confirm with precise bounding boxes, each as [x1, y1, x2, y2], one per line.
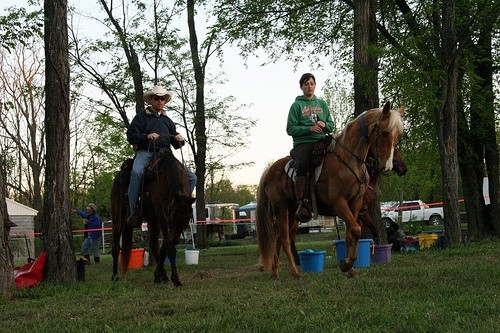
[71, 203, 102, 264]
[126, 86, 197, 223]
[286, 73, 334, 219]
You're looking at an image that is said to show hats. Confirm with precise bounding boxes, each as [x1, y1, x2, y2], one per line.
[89, 203, 98, 210]
[143, 86, 172, 102]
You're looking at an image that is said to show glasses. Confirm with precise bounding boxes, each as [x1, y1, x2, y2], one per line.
[151, 95, 166, 101]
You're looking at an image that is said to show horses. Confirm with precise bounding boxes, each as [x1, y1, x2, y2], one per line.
[254, 101, 407, 281]
[110, 156, 197, 287]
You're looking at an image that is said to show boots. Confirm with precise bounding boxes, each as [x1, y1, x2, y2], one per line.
[294, 175, 310, 222]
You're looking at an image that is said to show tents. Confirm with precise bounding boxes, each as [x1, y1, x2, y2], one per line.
[237, 203, 257, 211]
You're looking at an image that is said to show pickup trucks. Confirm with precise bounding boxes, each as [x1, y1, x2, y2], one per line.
[381, 198, 444, 230]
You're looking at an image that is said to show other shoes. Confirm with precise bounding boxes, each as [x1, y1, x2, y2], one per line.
[127, 213, 142, 225]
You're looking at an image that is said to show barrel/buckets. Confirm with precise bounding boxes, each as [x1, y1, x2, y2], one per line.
[371, 244, 393, 265]
[119, 249, 144, 268]
[397, 229, 444, 252]
[185, 250, 199, 264]
[298, 241, 327, 272]
[330, 239, 373, 268]
